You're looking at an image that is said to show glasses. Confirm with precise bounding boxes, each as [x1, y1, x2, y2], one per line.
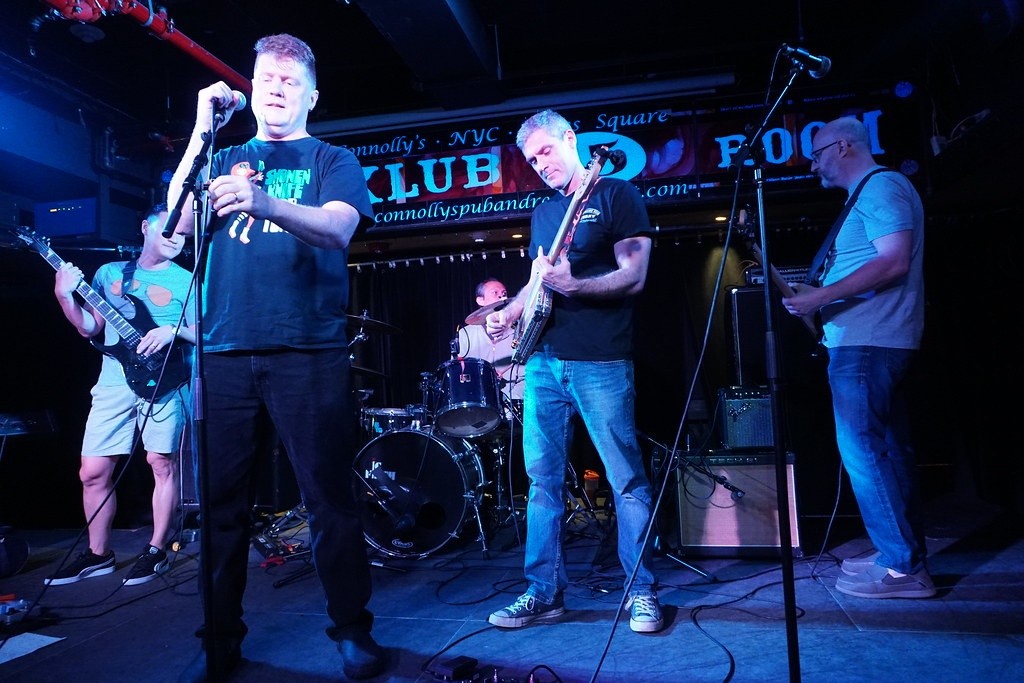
[809, 140, 852, 164]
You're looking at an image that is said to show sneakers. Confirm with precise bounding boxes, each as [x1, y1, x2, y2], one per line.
[43, 548, 117, 585]
[123, 544, 169, 585]
[625, 594, 664, 632]
[488, 594, 565, 627]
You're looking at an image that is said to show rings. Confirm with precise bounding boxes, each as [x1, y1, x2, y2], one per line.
[234, 192, 238, 202]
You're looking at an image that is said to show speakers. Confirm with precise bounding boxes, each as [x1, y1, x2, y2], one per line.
[671, 385, 807, 558]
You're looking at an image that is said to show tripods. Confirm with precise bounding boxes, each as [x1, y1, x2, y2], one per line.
[591, 435, 739, 581]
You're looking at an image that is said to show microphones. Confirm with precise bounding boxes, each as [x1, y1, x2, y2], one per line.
[782, 46, 832, 79]
[214, 90, 246, 111]
[730, 490, 744, 502]
[397, 515, 411, 530]
[454, 324, 460, 354]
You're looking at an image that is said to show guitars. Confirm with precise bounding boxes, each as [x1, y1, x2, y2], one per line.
[6, 223, 193, 403]
[732, 204, 830, 375]
[511, 144, 609, 366]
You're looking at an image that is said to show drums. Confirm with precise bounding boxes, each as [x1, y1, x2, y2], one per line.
[363, 407, 423, 442]
[351, 425, 485, 560]
[431, 356, 503, 438]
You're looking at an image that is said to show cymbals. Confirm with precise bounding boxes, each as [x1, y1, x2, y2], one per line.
[346, 313, 404, 336]
[349, 360, 386, 377]
[465, 297, 517, 325]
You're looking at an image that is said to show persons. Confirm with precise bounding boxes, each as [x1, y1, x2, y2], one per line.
[486, 111, 662, 632]
[166, 34, 382, 683]
[474, 279, 508, 308]
[44, 205, 195, 586]
[781, 118, 937, 599]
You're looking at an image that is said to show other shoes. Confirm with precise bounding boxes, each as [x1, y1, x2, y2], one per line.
[337, 633, 385, 681]
[205, 641, 241, 683]
[835, 565, 936, 598]
[840, 551, 884, 576]
